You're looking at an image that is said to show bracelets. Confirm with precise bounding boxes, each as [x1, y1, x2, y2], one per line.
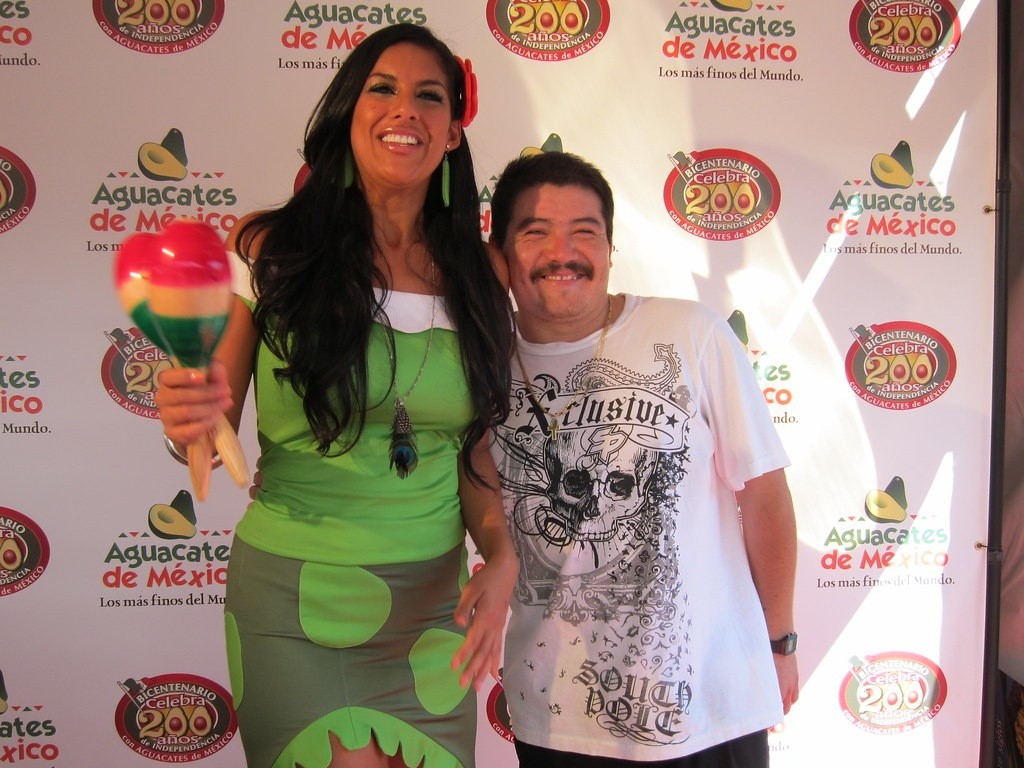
[163, 432, 224, 471]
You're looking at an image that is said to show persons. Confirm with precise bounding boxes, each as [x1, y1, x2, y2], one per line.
[488, 152, 797, 768]
[156, 22, 519, 768]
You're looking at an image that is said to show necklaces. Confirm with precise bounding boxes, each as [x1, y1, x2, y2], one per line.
[379, 254, 435, 481]
[513, 293, 613, 440]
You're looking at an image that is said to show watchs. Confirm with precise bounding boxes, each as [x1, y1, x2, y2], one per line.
[770, 633, 797, 656]
